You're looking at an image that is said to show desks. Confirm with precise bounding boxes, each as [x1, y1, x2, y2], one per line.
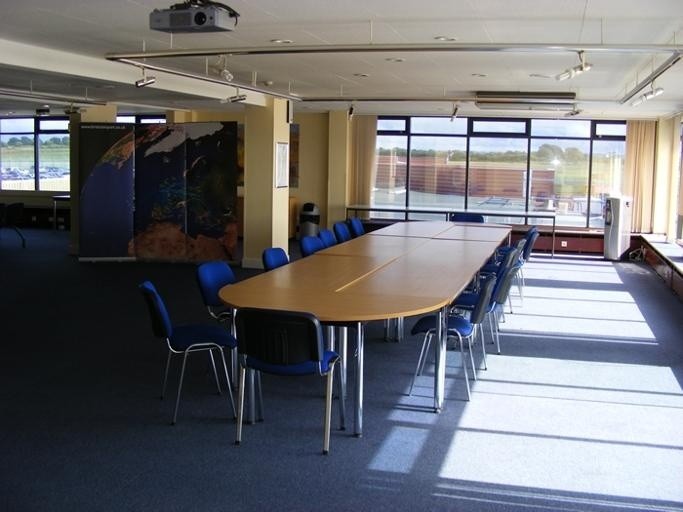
[345, 203, 556, 259]
[219, 219, 516, 437]
[50, 193, 70, 231]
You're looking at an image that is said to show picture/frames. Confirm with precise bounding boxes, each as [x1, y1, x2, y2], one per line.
[274, 141, 288, 188]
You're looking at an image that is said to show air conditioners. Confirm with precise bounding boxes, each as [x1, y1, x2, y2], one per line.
[603, 196, 633, 262]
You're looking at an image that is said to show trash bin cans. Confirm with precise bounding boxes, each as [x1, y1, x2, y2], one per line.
[300, 203, 320, 240]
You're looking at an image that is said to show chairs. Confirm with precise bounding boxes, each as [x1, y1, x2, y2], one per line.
[320, 230, 335, 248]
[262, 248, 288, 271]
[512, 238, 526, 263]
[478, 248, 517, 322]
[196, 258, 250, 335]
[229, 305, 347, 455]
[333, 222, 351, 243]
[137, 278, 238, 425]
[520, 231, 539, 259]
[446, 210, 482, 224]
[523, 226, 537, 249]
[407, 268, 498, 403]
[299, 236, 325, 258]
[451, 265, 496, 370]
[477, 261, 523, 354]
[350, 218, 365, 236]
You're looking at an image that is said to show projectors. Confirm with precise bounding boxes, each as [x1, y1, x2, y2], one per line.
[150, 0, 240, 32]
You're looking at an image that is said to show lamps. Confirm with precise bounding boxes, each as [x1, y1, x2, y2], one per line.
[209, 55, 239, 83]
[218, 82, 248, 108]
[554, 49, 593, 81]
[36, 103, 51, 116]
[449, 103, 464, 121]
[135, 62, 158, 89]
[629, 80, 663, 108]
